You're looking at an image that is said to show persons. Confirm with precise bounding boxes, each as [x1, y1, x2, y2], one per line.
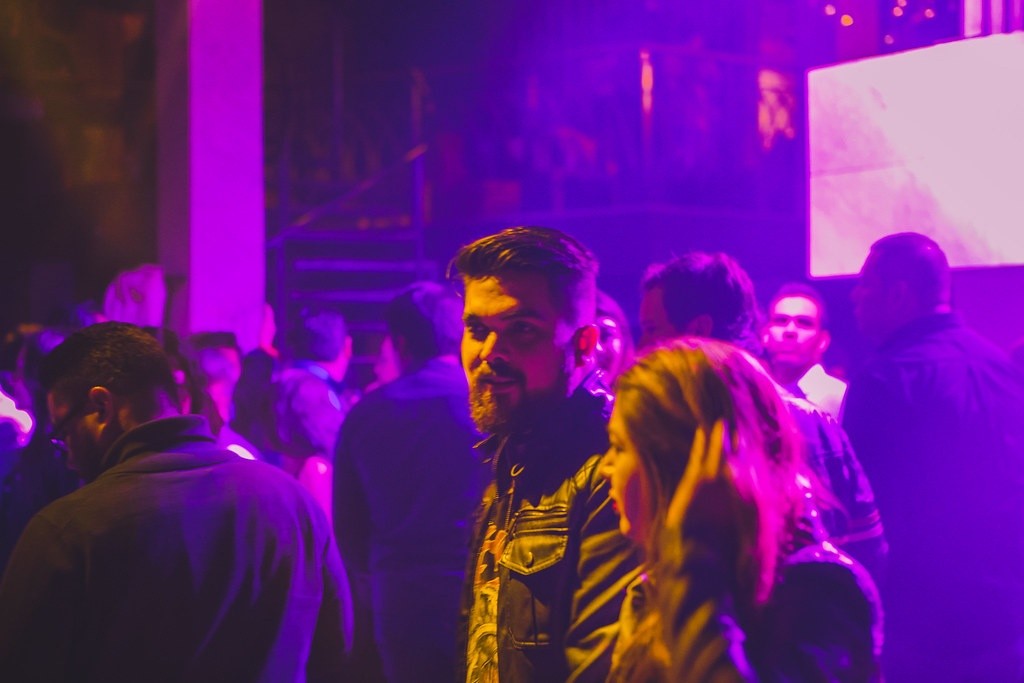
[1, 251, 497, 683]
[595, 251, 892, 610]
[602, 336, 887, 683]
[839, 231, 1023, 683]
[456, 226, 646, 683]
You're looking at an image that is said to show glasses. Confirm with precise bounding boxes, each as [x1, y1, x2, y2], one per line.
[49, 416, 70, 451]
